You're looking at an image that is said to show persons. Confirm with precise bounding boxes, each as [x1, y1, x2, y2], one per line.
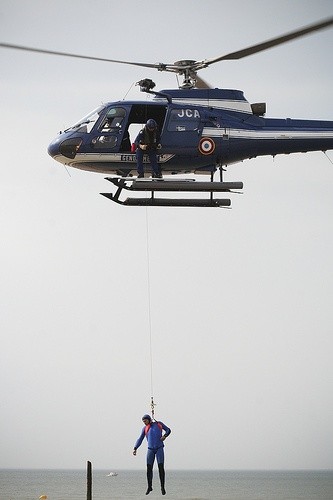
[132, 414, 171, 495]
[134, 119, 164, 182]
[104, 117, 120, 142]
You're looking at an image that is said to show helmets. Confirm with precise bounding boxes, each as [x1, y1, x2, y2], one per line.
[147, 119, 157, 128]
[142, 414, 151, 420]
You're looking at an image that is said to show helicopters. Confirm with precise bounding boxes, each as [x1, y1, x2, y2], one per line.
[1, 15, 333, 208]
